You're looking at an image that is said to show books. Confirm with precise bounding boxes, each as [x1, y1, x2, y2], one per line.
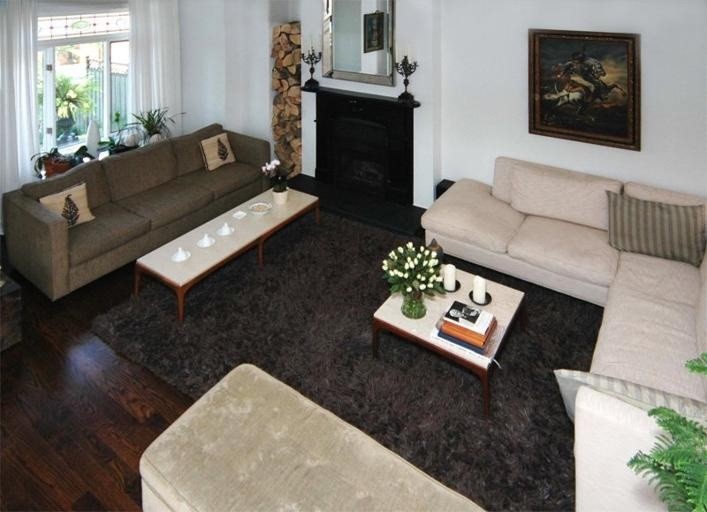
[435, 300, 497, 355]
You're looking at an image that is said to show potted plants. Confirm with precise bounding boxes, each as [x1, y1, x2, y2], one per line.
[125, 109, 185, 146]
[97, 111, 139, 155]
[31, 145, 95, 179]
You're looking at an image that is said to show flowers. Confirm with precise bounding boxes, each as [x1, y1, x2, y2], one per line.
[262, 159, 296, 192]
[383, 243, 445, 300]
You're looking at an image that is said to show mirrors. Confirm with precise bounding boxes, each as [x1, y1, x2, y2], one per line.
[322, 1, 396, 86]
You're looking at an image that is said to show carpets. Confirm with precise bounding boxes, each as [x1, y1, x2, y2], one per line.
[62, 208, 603, 512]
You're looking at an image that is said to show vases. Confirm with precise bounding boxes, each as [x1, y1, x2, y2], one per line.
[271, 187, 288, 205]
[402, 290, 427, 319]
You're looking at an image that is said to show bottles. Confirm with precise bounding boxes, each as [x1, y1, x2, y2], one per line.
[426, 239, 443, 276]
[85, 120, 100, 161]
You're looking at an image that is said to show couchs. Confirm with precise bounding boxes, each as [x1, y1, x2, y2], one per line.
[138, 158, 707, 512]
[4, 123, 270, 301]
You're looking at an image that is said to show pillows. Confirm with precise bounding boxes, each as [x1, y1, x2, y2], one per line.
[508, 166, 620, 230]
[606, 191, 707, 269]
[38, 182, 94, 229]
[554, 370, 707, 433]
[200, 133, 236, 171]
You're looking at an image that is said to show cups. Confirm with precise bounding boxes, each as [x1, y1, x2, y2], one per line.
[443, 264, 456, 291]
[472, 277, 486, 303]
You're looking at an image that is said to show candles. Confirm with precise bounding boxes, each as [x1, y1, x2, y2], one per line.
[474, 276, 486, 303]
[444, 264, 456, 290]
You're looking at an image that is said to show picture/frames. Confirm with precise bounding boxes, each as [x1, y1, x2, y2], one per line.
[364, 12, 384, 53]
[529, 28, 641, 152]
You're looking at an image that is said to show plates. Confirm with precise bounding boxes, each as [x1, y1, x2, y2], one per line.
[440, 280, 460, 292]
[468, 291, 491, 306]
[216, 228, 234, 236]
[171, 251, 191, 263]
[248, 201, 272, 215]
[196, 239, 215, 247]
